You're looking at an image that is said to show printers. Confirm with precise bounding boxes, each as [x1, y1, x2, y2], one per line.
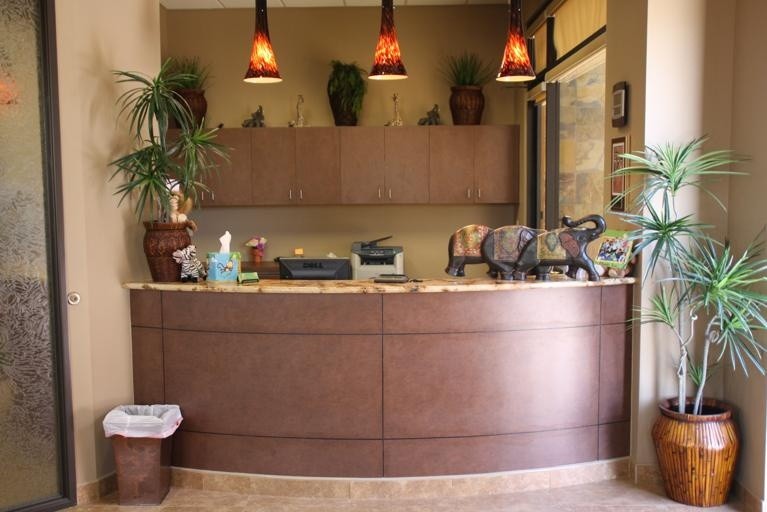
[350, 236, 404, 280]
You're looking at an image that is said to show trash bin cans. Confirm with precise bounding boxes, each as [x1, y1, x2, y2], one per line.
[102, 404, 180, 506]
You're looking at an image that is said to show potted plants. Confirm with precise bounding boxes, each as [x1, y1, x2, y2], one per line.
[106, 56, 236, 282]
[437, 50, 499, 125]
[596, 133, 767, 506]
[160, 52, 218, 128]
[326, 60, 368, 126]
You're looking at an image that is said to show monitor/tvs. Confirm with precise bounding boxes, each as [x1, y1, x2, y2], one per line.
[279, 258, 351, 280]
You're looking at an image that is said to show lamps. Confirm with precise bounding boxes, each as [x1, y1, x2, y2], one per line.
[368, 0, 409, 81]
[243, 0, 285, 84]
[495, 0, 538, 83]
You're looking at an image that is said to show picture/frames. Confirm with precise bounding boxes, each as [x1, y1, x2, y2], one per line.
[609, 135, 631, 214]
[611, 80, 629, 127]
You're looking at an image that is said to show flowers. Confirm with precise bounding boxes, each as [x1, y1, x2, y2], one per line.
[245, 237, 267, 252]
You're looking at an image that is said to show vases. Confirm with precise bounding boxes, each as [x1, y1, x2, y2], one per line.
[253, 248, 262, 263]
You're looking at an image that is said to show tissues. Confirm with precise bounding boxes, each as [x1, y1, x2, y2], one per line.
[207, 230, 241, 282]
